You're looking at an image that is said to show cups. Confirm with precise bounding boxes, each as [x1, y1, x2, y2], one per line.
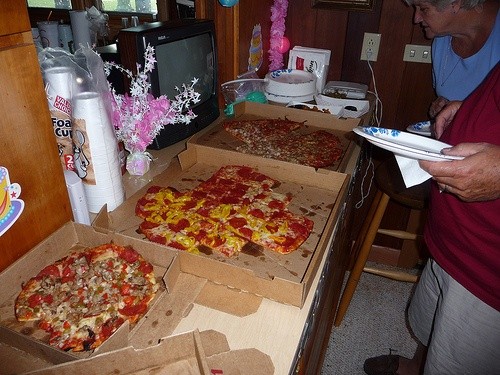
[30, 10, 138, 225]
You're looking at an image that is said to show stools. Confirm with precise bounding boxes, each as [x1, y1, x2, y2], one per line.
[335, 160, 430, 326]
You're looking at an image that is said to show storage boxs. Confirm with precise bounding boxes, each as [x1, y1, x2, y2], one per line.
[188, 101, 362, 185]
[0, 220, 181, 365]
[96, 143, 350, 308]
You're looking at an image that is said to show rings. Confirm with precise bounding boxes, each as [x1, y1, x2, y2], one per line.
[444, 185, 448, 193]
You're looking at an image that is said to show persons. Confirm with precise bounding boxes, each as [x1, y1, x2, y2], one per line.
[403, 0, 500, 120]
[364, 54, 500, 375]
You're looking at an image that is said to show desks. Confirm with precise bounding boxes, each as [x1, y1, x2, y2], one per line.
[161, 96, 376, 375]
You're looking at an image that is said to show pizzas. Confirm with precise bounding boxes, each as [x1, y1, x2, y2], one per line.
[235, 130, 342, 168]
[223, 118, 301, 143]
[15, 243, 159, 352]
[137, 164, 313, 258]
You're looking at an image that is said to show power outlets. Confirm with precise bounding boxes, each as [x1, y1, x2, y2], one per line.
[360, 33, 381, 62]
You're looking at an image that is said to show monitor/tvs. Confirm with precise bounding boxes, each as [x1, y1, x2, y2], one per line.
[96, 18, 220, 150]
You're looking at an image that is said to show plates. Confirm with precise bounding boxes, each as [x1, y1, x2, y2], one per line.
[406, 121, 434, 137]
[352, 126, 465, 161]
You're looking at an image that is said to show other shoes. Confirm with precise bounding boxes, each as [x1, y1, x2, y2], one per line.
[364, 354, 412, 375]
